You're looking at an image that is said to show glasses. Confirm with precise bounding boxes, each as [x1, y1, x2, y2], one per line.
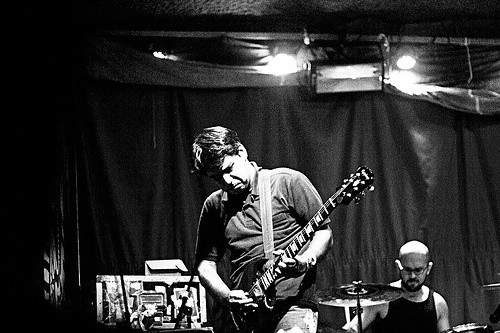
[401, 264, 429, 274]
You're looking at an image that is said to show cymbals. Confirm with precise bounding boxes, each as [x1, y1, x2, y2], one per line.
[309, 283, 406, 308]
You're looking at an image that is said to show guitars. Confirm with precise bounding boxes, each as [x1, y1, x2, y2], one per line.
[211, 164, 376, 333]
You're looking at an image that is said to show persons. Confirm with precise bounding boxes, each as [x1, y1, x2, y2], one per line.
[189, 126, 334, 333]
[343, 240, 451, 333]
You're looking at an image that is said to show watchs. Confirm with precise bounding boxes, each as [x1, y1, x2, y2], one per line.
[305, 251, 317, 268]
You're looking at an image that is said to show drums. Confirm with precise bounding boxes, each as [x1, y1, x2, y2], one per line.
[441, 321, 494, 333]
